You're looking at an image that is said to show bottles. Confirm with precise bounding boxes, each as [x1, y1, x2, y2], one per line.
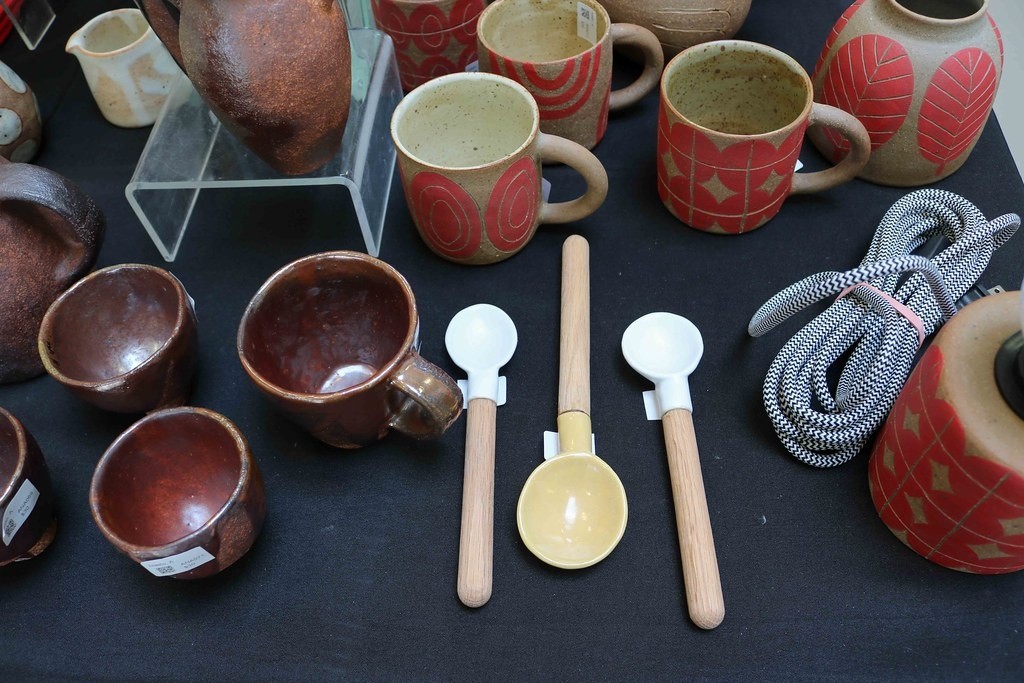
[808, 1, 1005, 189]
[869, 291, 1023, 578]
[175, 0, 355, 176]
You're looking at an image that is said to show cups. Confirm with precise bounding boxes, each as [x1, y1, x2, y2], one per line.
[387, 70, 607, 263]
[657, 40, 871, 238]
[371, 0, 485, 103]
[1, 157, 105, 385]
[36, 261, 205, 419]
[475, 3, 665, 153]
[236, 251, 464, 457]
[0, 408, 59, 567]
[88, 404, 265, 584]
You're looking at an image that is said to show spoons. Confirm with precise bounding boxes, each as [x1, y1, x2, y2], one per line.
[513, 225, 629, 568]
[620, 309, 727, 631]
[448, 298, 518, 607]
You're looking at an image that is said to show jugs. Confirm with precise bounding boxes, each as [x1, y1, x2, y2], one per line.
[67, 8, 193, 129]
